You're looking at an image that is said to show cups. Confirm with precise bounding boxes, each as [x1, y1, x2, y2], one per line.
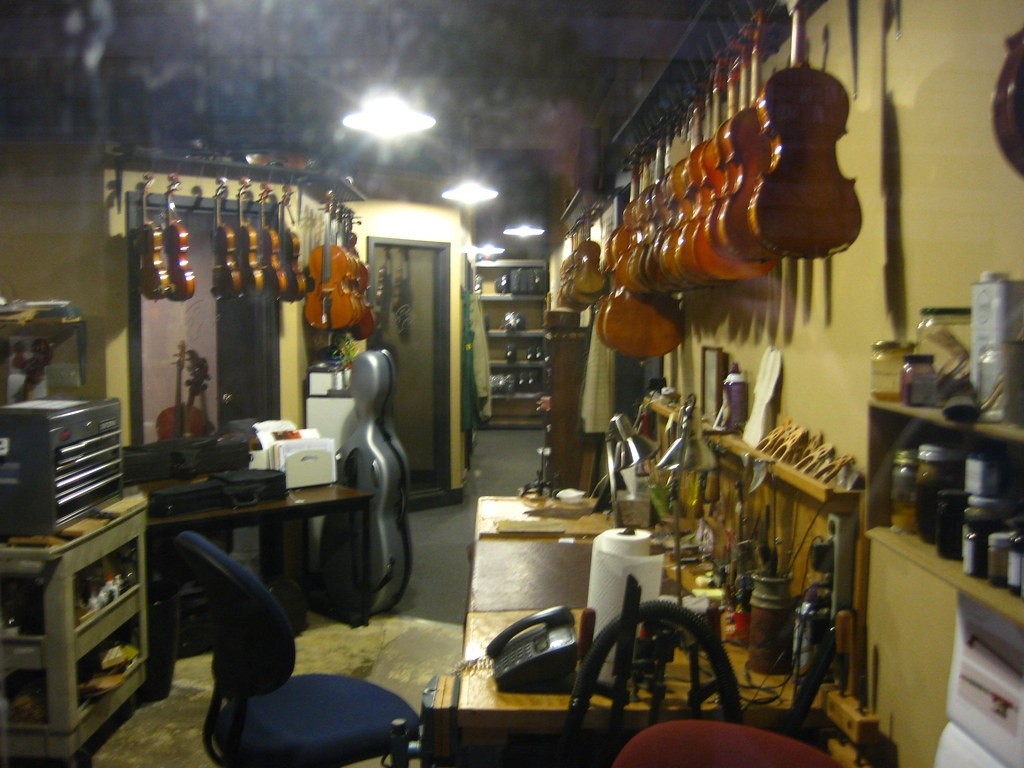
[989, 533, 1009, 587]
[934, 490, 968, 559]
[963, 507, 990, 576]
[873, 342, 915, 398]
[917, 306, 971, 395]
[750, 573, 794, 671]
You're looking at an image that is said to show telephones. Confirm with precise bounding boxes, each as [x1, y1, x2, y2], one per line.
[487, 605, 578, 693]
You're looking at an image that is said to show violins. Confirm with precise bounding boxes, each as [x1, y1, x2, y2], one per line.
[159, 176, 196, 302]
[138, 170, 169, 303]
[304, 190, 376, 342]
[210, 174, 309, 304]
[557, 0, 865, 311]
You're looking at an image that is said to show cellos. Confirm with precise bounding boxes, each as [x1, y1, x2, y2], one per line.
[593, 224, 619, 346]
[156, 338, 212, 442]
[594, 154, 687, 359]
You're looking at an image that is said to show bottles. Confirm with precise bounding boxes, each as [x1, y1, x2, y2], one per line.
[917, 443, 947, 478]
[900, 355, 939, 406]
[1008, 503, 1024, 594]
[724, 364, 747, 427]
[890, 451, 914, 533]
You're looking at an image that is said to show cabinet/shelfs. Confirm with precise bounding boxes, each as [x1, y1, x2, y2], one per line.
[854, 399, 1024, 768]
[0, 493, 152, 768]
[475, 260, 549, 429]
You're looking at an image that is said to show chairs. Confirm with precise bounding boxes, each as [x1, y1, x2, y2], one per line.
[176, 531, 422, 768]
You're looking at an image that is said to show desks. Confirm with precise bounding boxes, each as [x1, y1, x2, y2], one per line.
[136, 474, 377, 629]
